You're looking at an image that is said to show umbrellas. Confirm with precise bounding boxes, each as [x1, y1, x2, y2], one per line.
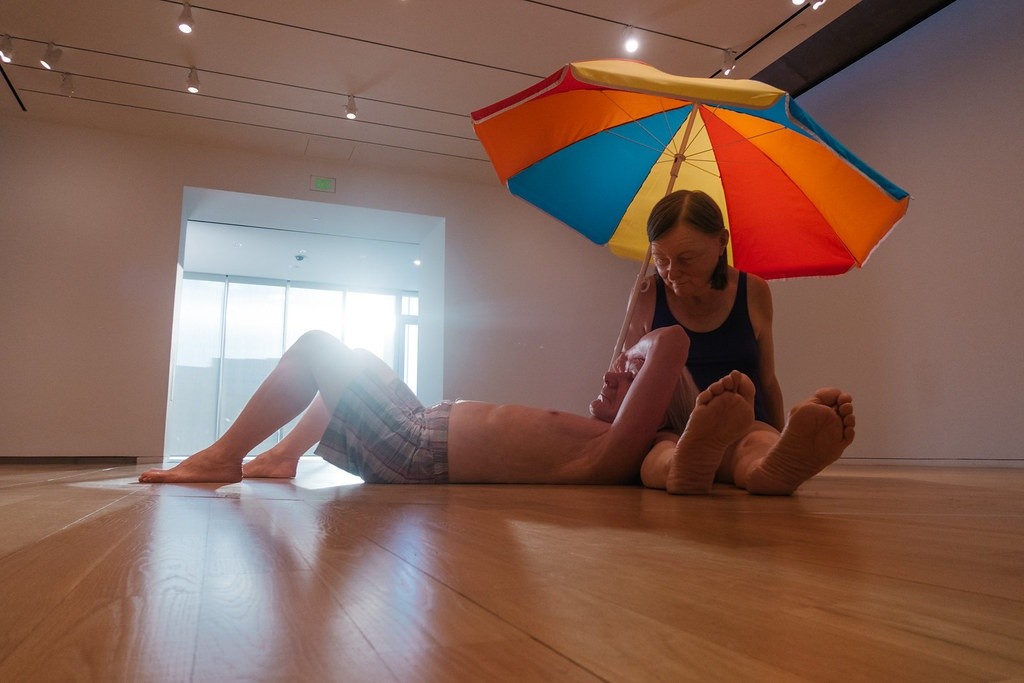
[469, 59, 912, 379]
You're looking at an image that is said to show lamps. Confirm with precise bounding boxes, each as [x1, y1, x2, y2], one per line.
[0, 0, 830, 127]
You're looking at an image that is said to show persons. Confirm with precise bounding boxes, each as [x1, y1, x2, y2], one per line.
[616, 188, 857, 499]
[141, 325, 703, 487]
